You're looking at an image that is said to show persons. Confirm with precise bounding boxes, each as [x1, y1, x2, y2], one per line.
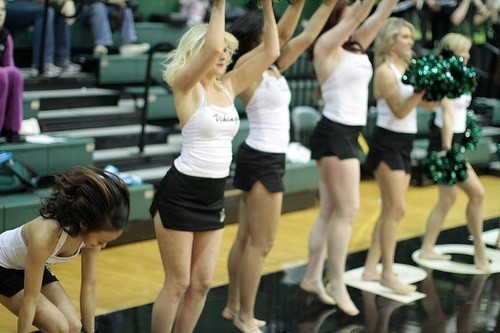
[0, 0, 500, 145]
[221, 0, 336, 333]
[149, 0, 281, 333]
[420, 32, 496, 272]
[0, 165, 131, 333]
[300, 0, 400, 316]
[363, 17, 440, 295]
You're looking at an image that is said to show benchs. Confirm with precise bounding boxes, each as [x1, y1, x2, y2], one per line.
[0, 0, 500, 250]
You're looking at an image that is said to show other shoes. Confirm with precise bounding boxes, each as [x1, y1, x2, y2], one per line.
[93, 44, 108, 58]
[4, 133, 25, 143]
[30, 62, 61, 77]
[55, 58, 81, 75]
[120, 42, 150, 57]
